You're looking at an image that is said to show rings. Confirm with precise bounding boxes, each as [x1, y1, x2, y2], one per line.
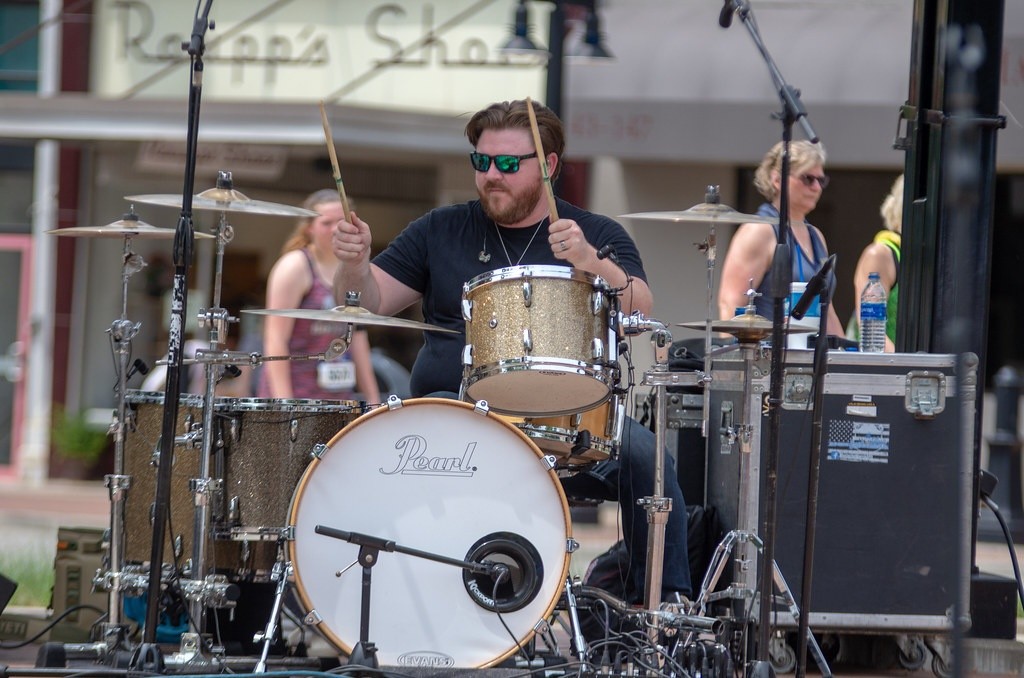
[560, 241, 567, 251]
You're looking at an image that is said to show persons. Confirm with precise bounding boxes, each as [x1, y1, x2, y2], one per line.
[719, 137, 846, 344]
[845, 173, 906, 354]
[332, 97, 693, 608]
[256, 188, 383, 407]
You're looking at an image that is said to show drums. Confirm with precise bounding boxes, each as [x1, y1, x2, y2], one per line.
[460, 263, 626, 420]
[495, 396, 613, 467]
[288, 397, 574, 670]
[109, 387, 359, 584]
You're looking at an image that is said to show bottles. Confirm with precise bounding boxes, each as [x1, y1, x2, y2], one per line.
[859, 272, 888, 353]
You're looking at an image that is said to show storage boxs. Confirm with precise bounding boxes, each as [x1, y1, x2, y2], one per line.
[701, 346, 978, 678]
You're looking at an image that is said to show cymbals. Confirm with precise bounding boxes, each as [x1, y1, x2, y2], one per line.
[240, 306, 462, 335]
[613, 203, 810, 227]
[42, 220, 216, 241]
[676, 313, 821, 343]
[122, 189, 324, 218]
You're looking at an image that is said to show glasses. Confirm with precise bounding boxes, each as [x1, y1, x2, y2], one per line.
[470, 151, 537, 174]
[789, 172, 830, 190]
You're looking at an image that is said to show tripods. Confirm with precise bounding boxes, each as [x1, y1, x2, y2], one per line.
[671, 340, 834, 678]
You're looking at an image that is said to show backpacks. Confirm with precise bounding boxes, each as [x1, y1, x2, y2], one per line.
[570, 504, 732, 667]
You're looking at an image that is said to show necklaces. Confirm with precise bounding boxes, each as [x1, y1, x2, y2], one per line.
[495, 212, 549, 268]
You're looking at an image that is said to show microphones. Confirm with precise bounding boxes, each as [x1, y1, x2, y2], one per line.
[492, 563, 511, 584]
[719, 0, 737, 29]
[619, 342, 635, 390]
[114, 358, 149, 391]
[216, 364, 242, 386]
[792, 254, 836, 321]
